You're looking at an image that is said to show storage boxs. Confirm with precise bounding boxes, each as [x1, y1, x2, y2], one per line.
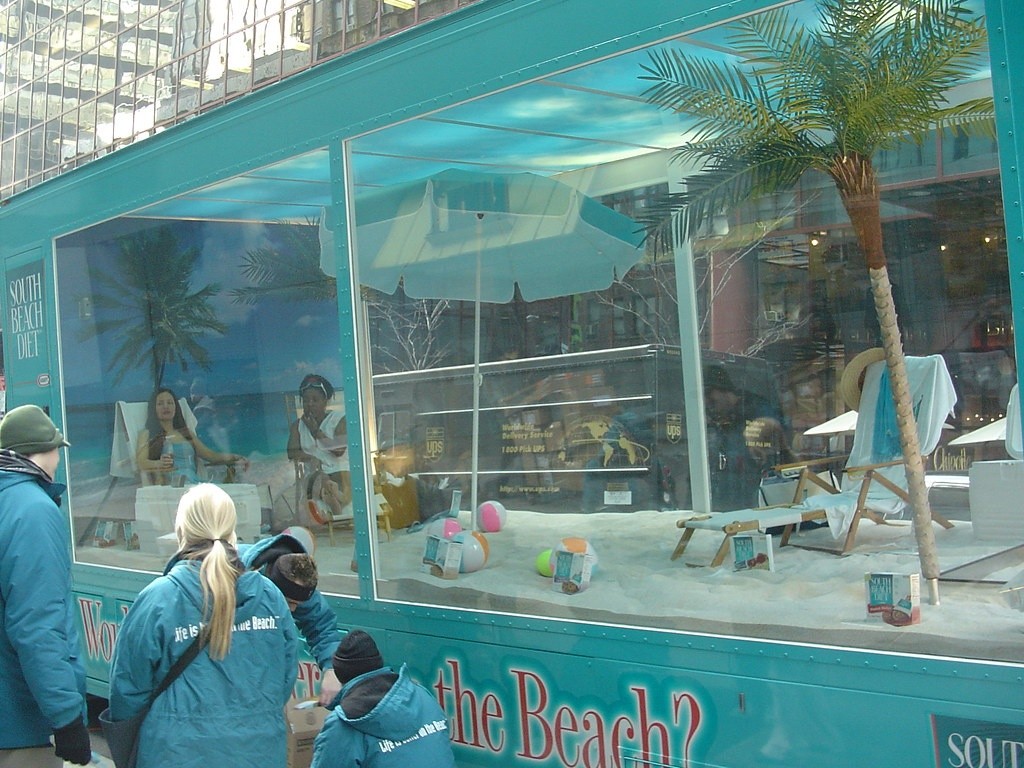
[286, 698, 332, 768]
[864, 570, 921, 627]
[421, 533, 465, 579]
[729, 534, 775, 574]
[552, 549, 595, 595]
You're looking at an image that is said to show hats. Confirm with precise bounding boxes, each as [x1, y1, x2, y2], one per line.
[0, 404, 72, 455]
[703, 364, 736, 391]
[333, 630, 383, 683]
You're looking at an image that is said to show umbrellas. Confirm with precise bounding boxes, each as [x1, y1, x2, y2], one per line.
[322, 162, 647, 531]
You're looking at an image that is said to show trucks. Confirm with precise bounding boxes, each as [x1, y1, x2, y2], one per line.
[372, 342, 782, 517]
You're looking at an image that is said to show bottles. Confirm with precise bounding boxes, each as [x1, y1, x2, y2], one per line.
[163, 440, 174, 464]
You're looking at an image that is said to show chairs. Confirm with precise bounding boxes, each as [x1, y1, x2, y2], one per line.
[282, 390, 394, 546]
[669, 354, 958, 568]
[109, 397, 276, 539]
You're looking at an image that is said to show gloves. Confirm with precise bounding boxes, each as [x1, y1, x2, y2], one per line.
[52, 712, 91, 765]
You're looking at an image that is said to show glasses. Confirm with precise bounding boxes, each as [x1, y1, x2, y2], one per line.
[300, 381, 327, 400]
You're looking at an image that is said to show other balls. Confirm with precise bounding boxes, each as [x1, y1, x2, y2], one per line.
[477, 499, 507, 533]
[428, 517, 462, 541]
[451, 529, 490, 573]
[536, 536, 600, 594]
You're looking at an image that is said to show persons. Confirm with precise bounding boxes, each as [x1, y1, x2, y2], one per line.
[238, 535, 342, 707]
[479, 336, 552, 503]
[310, 629, 455, 768]
[136, 389, 249, 484]
[0, 404, 91, 768]
[99, 482, 299, 768]
[288, 374, 351, 515]
